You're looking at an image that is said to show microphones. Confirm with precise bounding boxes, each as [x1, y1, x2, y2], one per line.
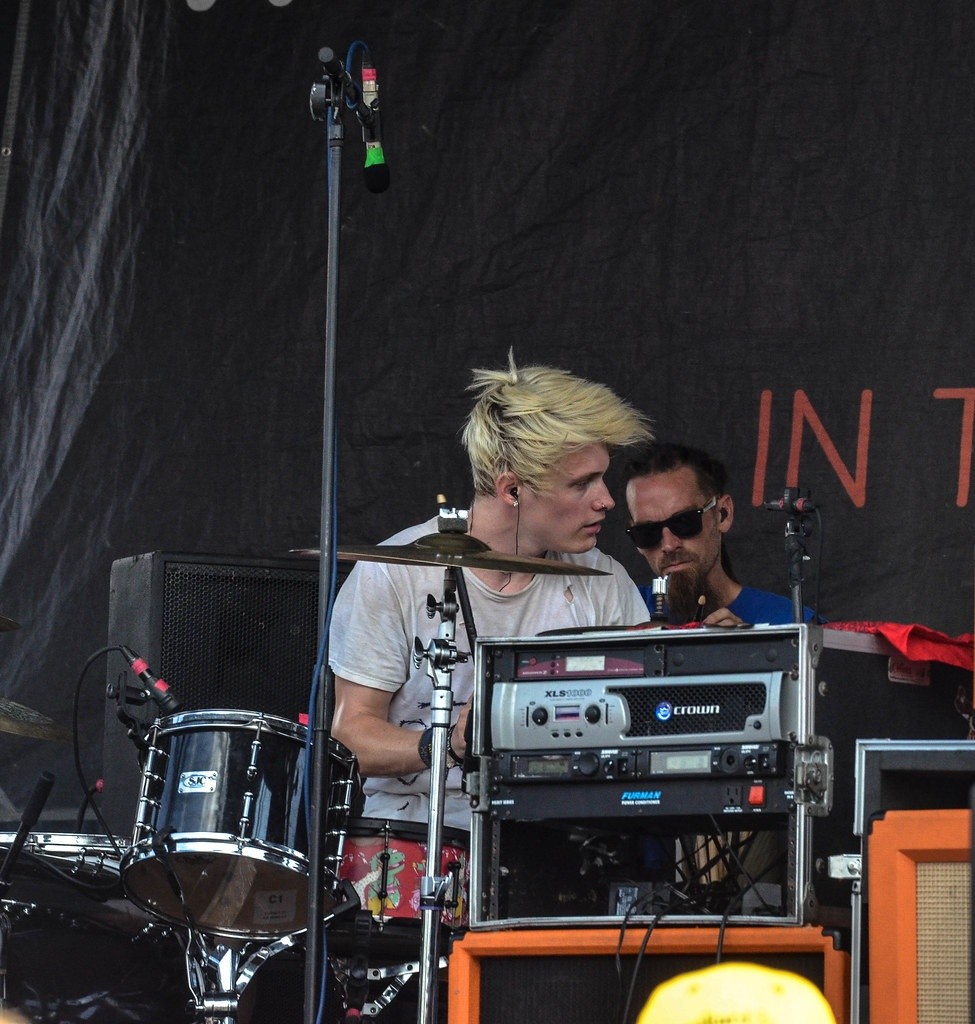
[360, 50, 390, 194]
[119, 646, 184, 714]
[765, 496, 821, 513]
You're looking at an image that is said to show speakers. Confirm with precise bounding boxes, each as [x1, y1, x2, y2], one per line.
[102, 549, 352, 842]
[448, 808, 971, 1024]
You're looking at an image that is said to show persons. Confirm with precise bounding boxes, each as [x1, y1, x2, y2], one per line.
[621, 446, 826, 628]
[326, 343, 656, 845]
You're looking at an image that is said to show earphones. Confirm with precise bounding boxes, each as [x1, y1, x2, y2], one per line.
[511, 487, 518, 500]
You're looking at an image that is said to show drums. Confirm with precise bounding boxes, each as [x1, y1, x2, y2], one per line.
[115, 704, 477, 968]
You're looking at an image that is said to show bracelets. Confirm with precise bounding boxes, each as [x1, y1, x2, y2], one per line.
[419, 726, 463, 772]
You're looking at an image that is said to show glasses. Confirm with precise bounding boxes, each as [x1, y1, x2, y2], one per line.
[624, 490, 721, 550]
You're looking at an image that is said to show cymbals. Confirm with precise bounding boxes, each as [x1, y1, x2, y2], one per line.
[287, 531, 618, 580]
[535, 621, 672, 636]
